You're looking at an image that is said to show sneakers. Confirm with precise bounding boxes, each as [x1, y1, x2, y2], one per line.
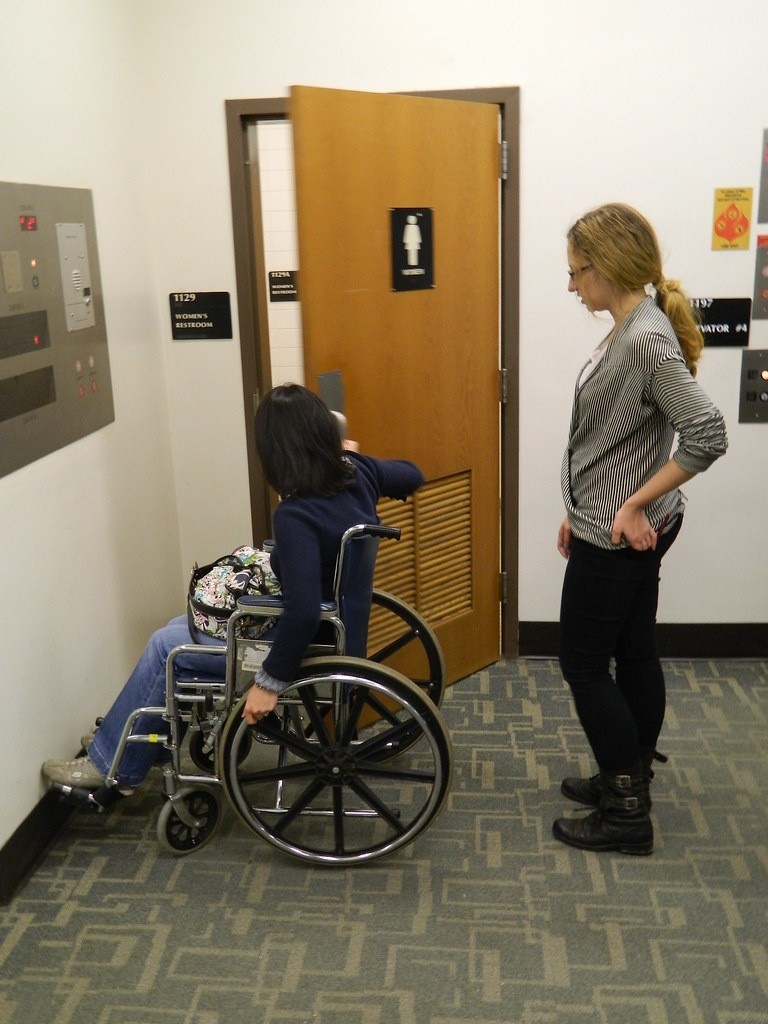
[42, 754, 110, 787]
[80, 733, 95, 750]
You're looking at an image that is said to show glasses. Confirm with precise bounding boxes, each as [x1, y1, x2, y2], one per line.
[567, 263, 593, 281]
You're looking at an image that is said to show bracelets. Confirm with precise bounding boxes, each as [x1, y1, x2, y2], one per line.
[254, 683, 277, 692]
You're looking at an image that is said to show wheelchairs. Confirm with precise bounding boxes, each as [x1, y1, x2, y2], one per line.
[52, 495, 456, 867]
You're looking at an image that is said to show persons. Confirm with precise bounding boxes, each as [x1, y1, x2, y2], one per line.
[40, 384, 422, 799]
[550, 202, 729, 852]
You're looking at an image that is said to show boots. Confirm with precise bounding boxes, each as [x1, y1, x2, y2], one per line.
[552, 772, 654, 854]
[561, 772, 606, 808]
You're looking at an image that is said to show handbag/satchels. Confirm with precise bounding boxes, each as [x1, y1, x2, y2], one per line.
[187, 545, 282, 641]
